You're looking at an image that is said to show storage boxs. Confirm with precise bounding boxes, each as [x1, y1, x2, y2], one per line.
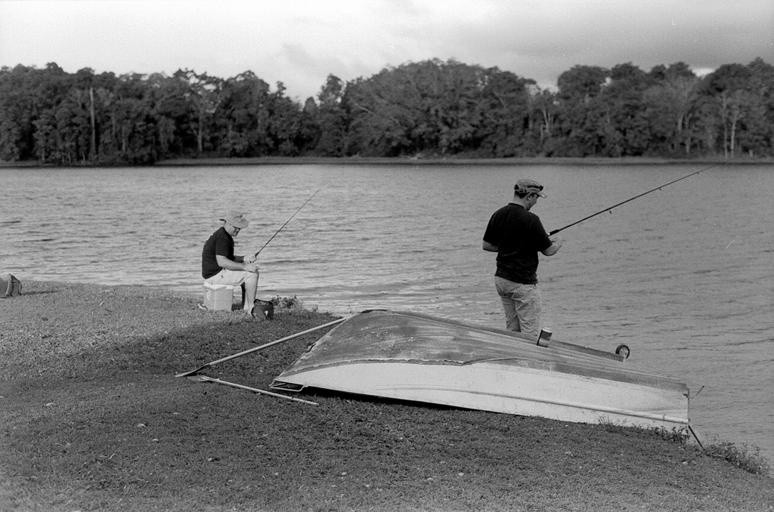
[202, 282, 233, 313]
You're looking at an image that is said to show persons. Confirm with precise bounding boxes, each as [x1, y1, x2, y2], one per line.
[616, 344, 630, 359]
[202, 209, 261, 314]
[481, 178, 562, 338]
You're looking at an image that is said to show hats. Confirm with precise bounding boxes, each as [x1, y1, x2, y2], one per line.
[515, 178, 547, 199]
[220, 211, 249, 228]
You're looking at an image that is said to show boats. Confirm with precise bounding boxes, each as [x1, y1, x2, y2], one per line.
[270, 304, 692, 439]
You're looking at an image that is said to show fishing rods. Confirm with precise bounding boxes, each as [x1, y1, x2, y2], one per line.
[547, 165, 716, 236]
[250, 190, 320, 263]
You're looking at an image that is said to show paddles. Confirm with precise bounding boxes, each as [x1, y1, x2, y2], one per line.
[187, 375, 319, 406]
[175, 314, 360, 378]
